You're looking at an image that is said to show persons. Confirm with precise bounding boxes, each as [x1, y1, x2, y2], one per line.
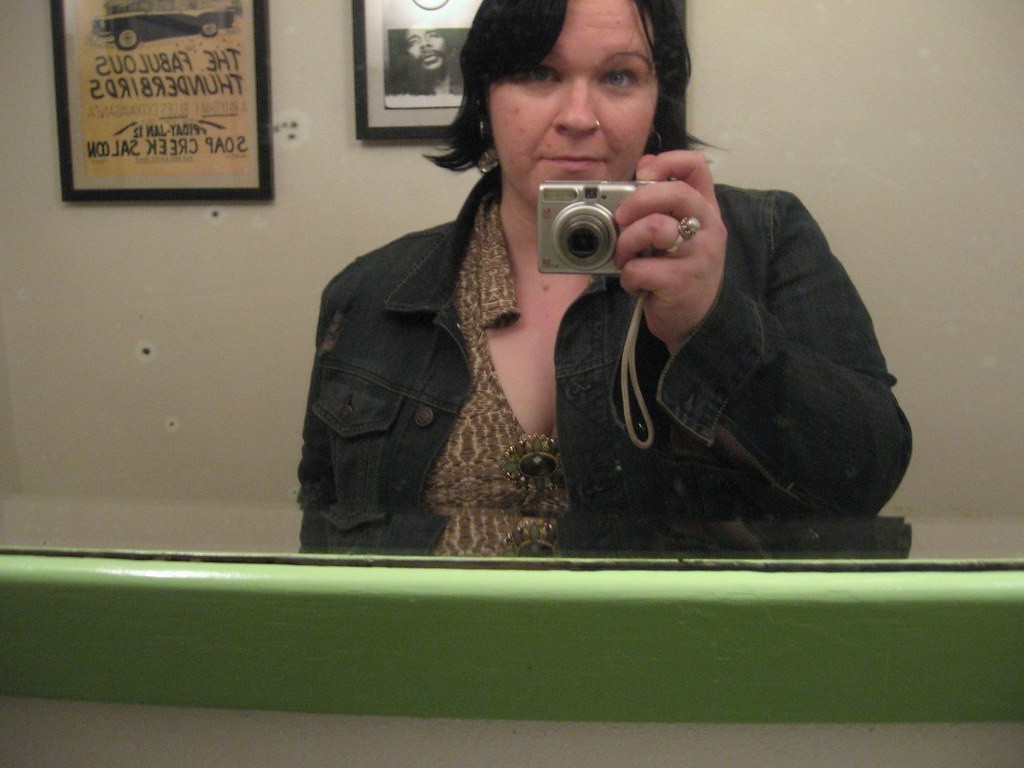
[295, 0, 914, 559]
[390, 26, 465, 95]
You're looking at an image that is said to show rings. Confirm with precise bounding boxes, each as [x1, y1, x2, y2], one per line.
[666, 215, 701, 255]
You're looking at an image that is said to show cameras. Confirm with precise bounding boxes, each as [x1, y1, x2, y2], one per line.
[535, 179, 663, 274]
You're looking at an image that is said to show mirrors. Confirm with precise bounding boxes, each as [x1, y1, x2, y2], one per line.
[1, 0, 1024, 573]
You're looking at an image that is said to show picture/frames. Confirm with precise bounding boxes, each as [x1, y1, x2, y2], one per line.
[353, 0, 488, 138]
[49, 0, 275, 200]
